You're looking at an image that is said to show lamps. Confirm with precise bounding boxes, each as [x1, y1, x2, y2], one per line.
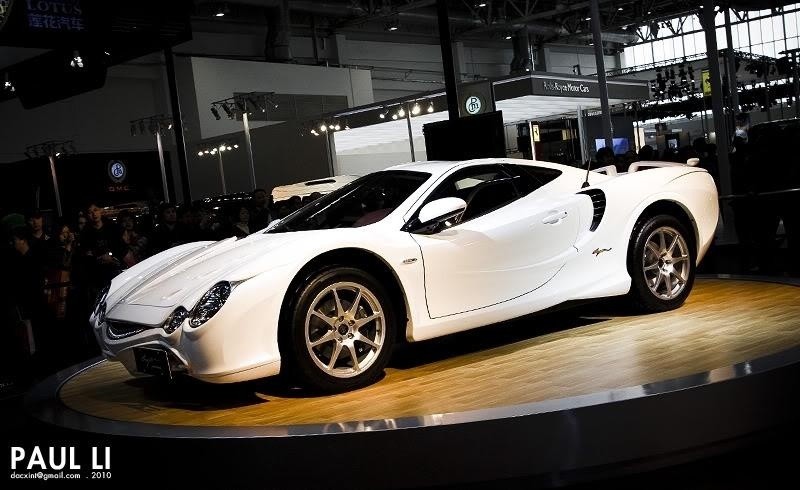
[380, 98, 435, 120]
[25, 140, 77, 160]
[213, 91, 277, 120]
[611, 52, 799, 121]
[197, 137, 239, 156]
[301, 113, 351, 137]
[130, 114, 172, 136]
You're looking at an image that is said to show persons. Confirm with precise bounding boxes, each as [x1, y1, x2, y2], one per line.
[1, 187, 323, 380]
[588, 119, 800, 244]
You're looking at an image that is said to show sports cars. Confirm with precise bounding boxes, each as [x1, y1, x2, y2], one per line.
[87, 159, 721, 396]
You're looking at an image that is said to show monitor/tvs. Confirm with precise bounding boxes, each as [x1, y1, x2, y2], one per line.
[594, 137, 630, 155]
